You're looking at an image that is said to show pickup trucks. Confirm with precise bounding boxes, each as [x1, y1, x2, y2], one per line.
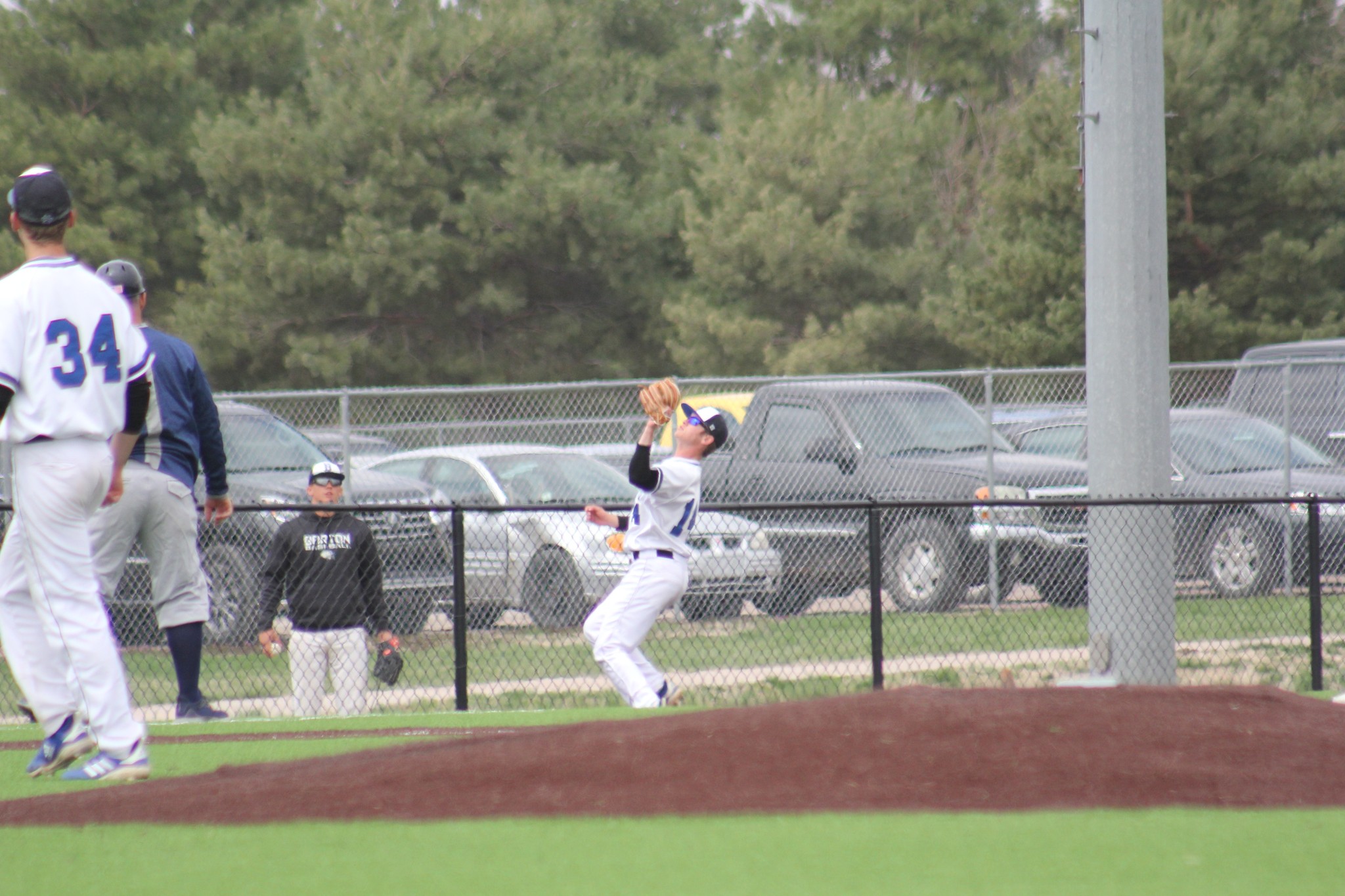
[700, 378, 1091, 611]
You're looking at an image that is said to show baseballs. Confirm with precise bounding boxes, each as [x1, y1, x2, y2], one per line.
[263, 642, 281, 658]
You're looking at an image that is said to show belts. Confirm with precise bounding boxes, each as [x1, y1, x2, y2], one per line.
[633, 550, 673, 559]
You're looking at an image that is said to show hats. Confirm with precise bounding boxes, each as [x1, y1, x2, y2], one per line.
[309, 461, 345, 479]
[681, 403, 728, 448]
[7, 167, 70, 222]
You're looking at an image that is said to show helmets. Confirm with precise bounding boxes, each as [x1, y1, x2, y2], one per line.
[95, 260, 145, 297]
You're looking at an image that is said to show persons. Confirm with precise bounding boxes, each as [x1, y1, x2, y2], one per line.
[257, 459, 393, 718]
[15, 262, 232, 722]
[0, 164, 152, 780]
[582, 403, 728, 708]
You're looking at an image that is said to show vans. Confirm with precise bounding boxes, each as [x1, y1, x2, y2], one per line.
[1224, 338, 1345, 468]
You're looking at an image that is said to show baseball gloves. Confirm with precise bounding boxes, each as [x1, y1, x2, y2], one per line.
[637, 379, 682, 427]
[372, 636, 404, 688]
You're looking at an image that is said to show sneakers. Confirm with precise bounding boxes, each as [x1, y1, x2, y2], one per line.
[175, 696, 228, 719]
[25, 713, 96, 777]
[59, 737, 150, 781]
[660, 679, 683, 706]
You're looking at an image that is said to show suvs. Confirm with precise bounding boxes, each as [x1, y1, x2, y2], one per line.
[101, 398, 455, 645]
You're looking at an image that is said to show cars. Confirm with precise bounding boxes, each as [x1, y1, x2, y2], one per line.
[568, 443, 666, 483]
[352, 442, 783, 633]
[302, 431, 398, 471]
[1004, 410, 1345, 596]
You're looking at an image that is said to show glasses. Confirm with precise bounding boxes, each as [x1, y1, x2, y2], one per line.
[310, 476, 343, 486]
[688, 416, 711, 435]
